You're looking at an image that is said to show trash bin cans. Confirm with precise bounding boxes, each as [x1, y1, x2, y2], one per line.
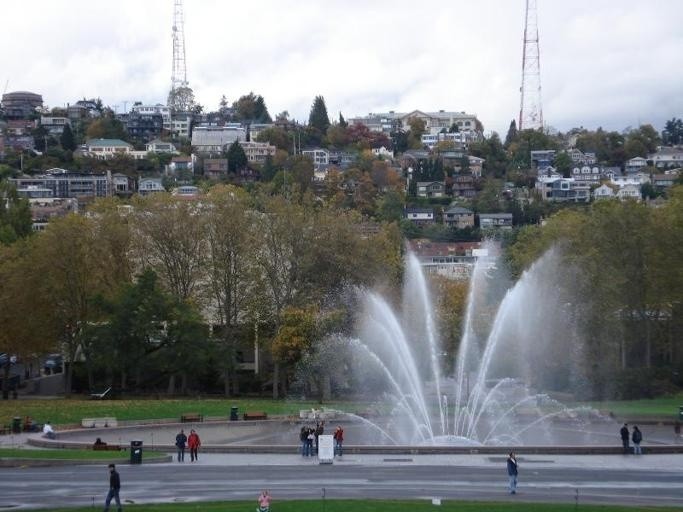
[131, 440, 143, 463]
[679, 406, 683, 419]
[231, 407, 239, 420]
[12, 416, 22, 433]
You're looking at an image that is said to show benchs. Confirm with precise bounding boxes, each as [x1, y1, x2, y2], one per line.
[181, 412, 203, 422]
[244, 410, 267, 420]
[93, 445, 122, 452]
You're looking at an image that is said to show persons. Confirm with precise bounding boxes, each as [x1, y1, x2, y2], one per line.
[104, 463, 123, 511]
[188, 428, 201, 464]
[174, 429, 187, 464]
[255, 490, 272, 511]
[631, 424, 643, 455]
[43, 421, 54, 439]
[505, 451, 519, 495]
[299, 420, 343, 458]
[619, 421, 630, 457]
